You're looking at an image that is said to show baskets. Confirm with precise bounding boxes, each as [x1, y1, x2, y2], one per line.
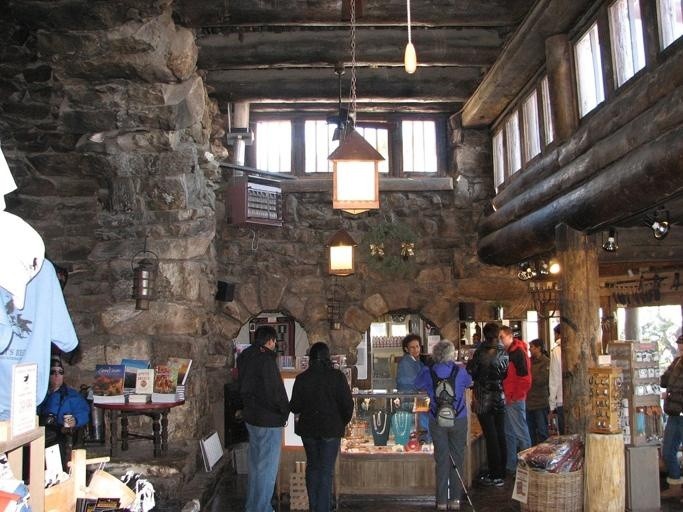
[517, 446, 584, 512]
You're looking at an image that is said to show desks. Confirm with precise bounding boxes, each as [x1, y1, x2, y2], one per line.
[93, 400, 185, 459]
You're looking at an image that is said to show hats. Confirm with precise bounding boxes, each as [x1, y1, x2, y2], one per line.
[554, 324, 560, 333]
[0, 211, 45, 309]
[676, 335, 683, 343]
[483, 322, 500, 337]
[310, 342, 330, 360]
[432, 339, 455, 362]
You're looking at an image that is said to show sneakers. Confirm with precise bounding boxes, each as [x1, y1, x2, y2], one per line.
[437, 503, 447, 509]
[448, 500, 460, 510]
[478, 471, 505, 486]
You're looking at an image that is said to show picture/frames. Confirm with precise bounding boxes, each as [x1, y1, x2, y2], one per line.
[249, 316, 296, 370]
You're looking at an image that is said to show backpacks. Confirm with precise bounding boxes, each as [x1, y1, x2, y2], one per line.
[429, 364, 464, 427]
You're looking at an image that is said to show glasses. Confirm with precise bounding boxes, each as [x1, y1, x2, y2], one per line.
[50, 370, 64, 376]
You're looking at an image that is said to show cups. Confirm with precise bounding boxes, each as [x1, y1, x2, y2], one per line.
[64, 413, 72, 428]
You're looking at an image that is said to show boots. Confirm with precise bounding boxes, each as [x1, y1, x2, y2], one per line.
[661, 477, 683, 497]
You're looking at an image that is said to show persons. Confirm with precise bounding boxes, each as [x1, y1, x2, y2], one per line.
[549, 323, 564, 436]
[466, 322, 507, 487]
[460, 321, 481, 348]
[291, 340, 354, 512]
[22, 359, 90, 486]
[527, 338, 550, 446]
[498, 326, 533, 472]
[416, 338, 473, 511]
[394, 334, 425, 392]
[237, 325, 290, 512]
[660, 335, 683, 500]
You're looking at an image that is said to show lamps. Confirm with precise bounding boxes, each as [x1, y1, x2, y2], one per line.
[642, 213, 671, 240]
[327, 0, 385, 216]
[326, 229, 358, 277]
[131, 237, 160, 310]
[404, 0, 417, 74]
[601, 227, 619, 253]
[548, 255, 561, 275]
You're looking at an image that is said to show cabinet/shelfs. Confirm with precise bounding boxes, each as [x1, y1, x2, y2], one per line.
[587, 364, 625, 435]
[331, 389, 468, 510]
[458, 319, 527, 364]
[607, 340, 664, 446]
[465, 388, 489, 489]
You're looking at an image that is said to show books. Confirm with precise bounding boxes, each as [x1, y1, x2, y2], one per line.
[92, 356, 193, 403]
